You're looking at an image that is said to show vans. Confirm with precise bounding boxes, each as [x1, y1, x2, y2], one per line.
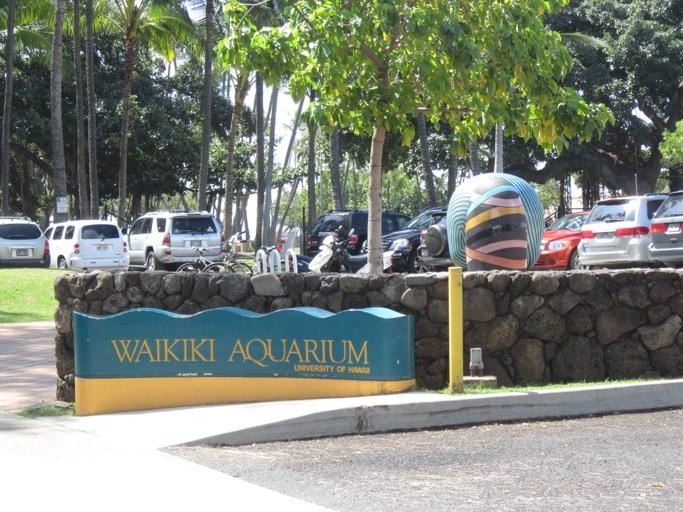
[45, 220, 130, 272]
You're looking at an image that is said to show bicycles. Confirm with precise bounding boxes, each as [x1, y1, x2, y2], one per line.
[175, 230, 302, 274]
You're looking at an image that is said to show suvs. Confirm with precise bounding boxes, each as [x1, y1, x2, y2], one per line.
[0, 216, 51, 268]
[121, 209, 226, 273]
